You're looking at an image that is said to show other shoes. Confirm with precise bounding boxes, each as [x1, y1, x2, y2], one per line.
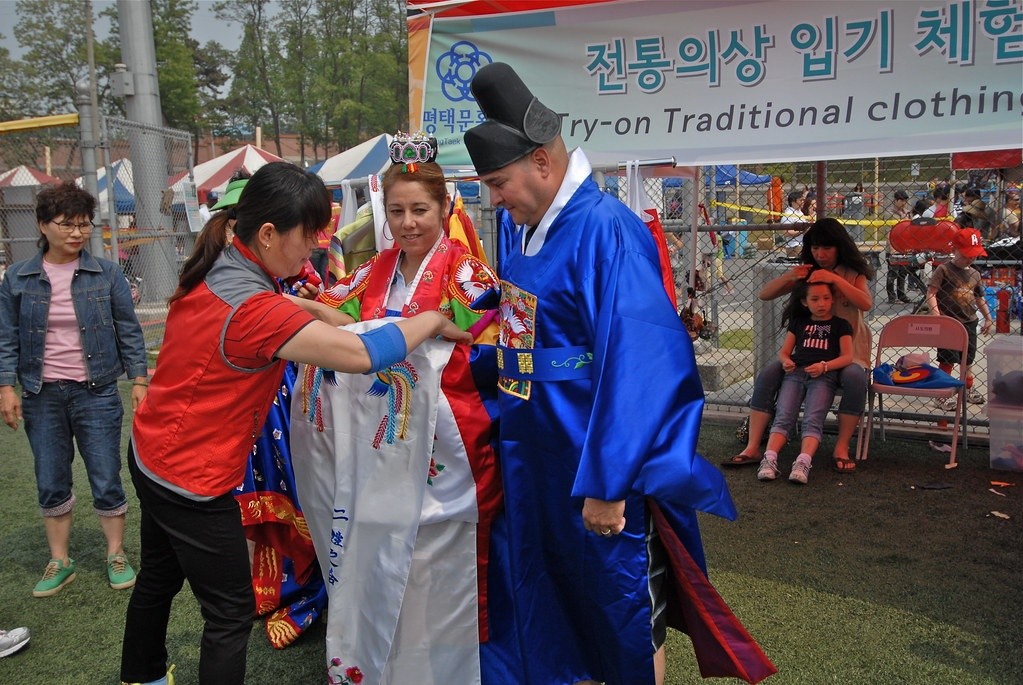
[889, 299, 895, 304]
[899, 296, 912, 304]
[723, 289, 734, 296]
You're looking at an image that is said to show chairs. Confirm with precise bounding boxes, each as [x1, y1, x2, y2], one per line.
[856, 318, 874, 460]
[863, 314, 969, 467]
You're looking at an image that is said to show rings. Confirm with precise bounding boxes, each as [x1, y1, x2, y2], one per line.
[601, 529, 611, 535]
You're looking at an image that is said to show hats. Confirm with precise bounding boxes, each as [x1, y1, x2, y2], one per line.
[463, 62, 561, 177]
[208, 192, 219, 199]
[953, 228, 988, 258]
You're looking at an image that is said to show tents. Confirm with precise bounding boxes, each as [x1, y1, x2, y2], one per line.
[0, 133, 398, 216]
[662, 164, 775, 252]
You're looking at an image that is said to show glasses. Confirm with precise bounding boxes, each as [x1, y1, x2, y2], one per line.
[52, 221, 96, 234]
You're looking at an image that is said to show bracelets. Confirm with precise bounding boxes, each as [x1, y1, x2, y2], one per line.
[985, 319, 993, 324]
[820, 360, 827, 374]
[133, 381, 148, 387]
[672, 239, 678, 244]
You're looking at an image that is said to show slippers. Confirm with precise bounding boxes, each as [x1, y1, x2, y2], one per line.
[830, 455, 857, 473]
[721, 455, 761, 465]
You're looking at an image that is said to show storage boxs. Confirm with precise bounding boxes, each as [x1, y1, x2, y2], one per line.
[981, 336, 1023, 473]
[984, 286, 1019, 320]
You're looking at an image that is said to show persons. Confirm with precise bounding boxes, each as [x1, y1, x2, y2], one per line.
[906, 175, 1023, 291]
[779, 184, 817, 259]
[0, 626, 31, 658]
[120, 161, 472, 685]
[854, 183, 864, 192]
[288, 131, 502, 685]
[209, 168, 329, 650]
[198, 191, 222, 230]
[665, 192, 735, 310]
[883, 190, 917, 304]
[925, 228, 993, 411]
[0, 180, 151, 596]
[731, 218, 873, 485]
[463, 63, 740, 685]
[768, 176, 785, 223]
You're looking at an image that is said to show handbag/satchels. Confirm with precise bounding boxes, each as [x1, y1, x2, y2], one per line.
[735, 416, 750, 443]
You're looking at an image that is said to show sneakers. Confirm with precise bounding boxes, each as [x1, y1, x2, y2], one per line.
[33, 557, 76, 596]
[1, 627, 31, 659]
[966, 386, 984, 404]
[107, 554, 137, 590]
[757, 455, 781, 480]
[934, 396, 957, 411]
[789, 459, 812, 484]
